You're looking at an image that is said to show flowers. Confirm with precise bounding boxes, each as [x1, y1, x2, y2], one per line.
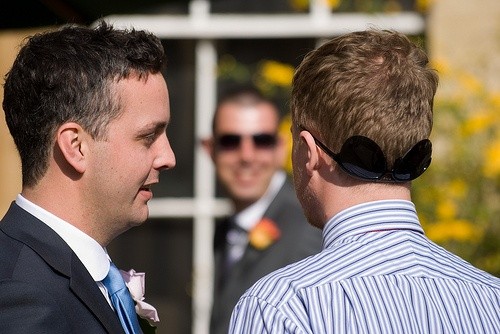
[119, 267, 161, 328]
[247, 218, 280, 251]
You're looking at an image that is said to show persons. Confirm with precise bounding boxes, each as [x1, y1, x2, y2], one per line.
[204, 81, 325, 334]
[224, 27, 499, 334]
[0, 23, 180, 334]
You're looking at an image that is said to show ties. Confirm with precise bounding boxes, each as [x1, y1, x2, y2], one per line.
[96, 260, 144, 334]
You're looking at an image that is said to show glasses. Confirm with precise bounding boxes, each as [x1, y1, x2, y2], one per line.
[210, 132, 279, 154]
[297, 124, 434, 185]
